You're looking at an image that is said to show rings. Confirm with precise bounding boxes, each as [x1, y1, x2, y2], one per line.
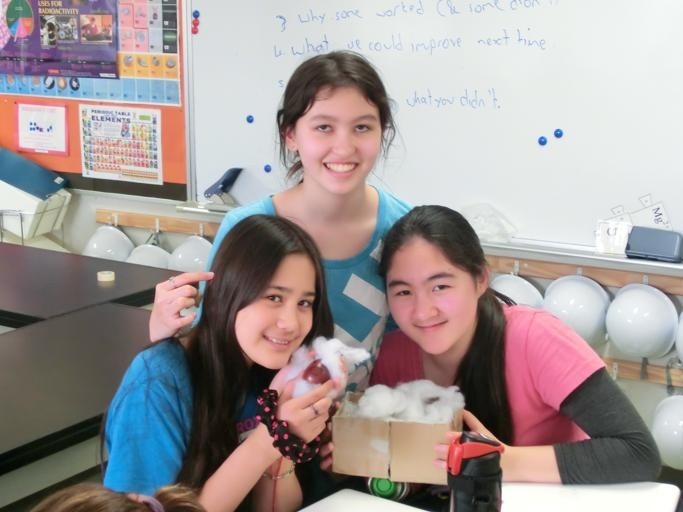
[310, 405, 320, 417]
[168, 276, 177, 289]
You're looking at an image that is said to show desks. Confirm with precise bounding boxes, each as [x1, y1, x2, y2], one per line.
[0, 302, 193, 511]
[0, 241, 198, 328]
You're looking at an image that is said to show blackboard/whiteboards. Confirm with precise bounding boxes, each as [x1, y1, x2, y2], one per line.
[189, 0, 683, 279]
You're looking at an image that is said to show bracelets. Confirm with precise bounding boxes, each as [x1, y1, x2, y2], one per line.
[262, 458, 297, 480]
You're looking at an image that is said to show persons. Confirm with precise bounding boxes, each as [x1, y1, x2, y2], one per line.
[147, 51, 417, 512]
[28, 480, 209, 512]
[366, 205, 662, 511]
[81, 16, 111, 41]
[101, 214, 334, 512]
[40, 16, 77, 48]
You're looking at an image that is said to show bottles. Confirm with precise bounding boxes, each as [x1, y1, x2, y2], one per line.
[446, 430, 505, 512]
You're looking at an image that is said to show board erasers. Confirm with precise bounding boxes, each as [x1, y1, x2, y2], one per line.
[624, 227, 683, 264]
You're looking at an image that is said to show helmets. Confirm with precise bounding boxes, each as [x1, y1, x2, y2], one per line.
[489, 274, 683, 368]
[82, 226, 213, 273]
[651, 395, 682, 471]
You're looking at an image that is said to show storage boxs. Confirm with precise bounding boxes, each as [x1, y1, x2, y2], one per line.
[327, 391, 464, 483]
[0, 147, 72, 238]
